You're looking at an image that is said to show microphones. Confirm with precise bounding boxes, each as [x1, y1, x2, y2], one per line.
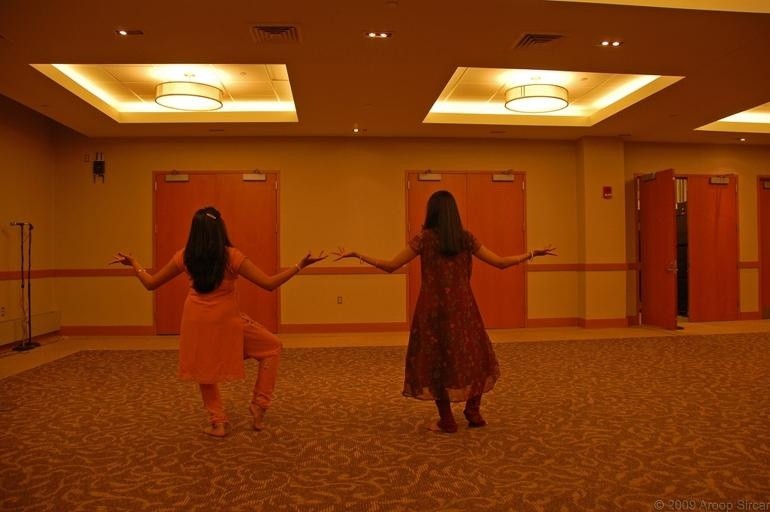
[10, 222, 24, 226]
[24, 222, 31, 225]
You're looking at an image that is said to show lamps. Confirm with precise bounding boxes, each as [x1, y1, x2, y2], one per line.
[155, 83, 223, 111]
[504, 84, 569, 113]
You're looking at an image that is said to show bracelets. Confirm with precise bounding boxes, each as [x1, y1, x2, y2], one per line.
[135, 268, 146, 275]
[530, 249, 534, 264]
[359, 254, 365, 264]
[295, 264, 303, 273]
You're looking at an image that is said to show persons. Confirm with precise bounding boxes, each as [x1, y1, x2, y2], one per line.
[106, 204, 330, 436]
[328, 190, 558, 433]
